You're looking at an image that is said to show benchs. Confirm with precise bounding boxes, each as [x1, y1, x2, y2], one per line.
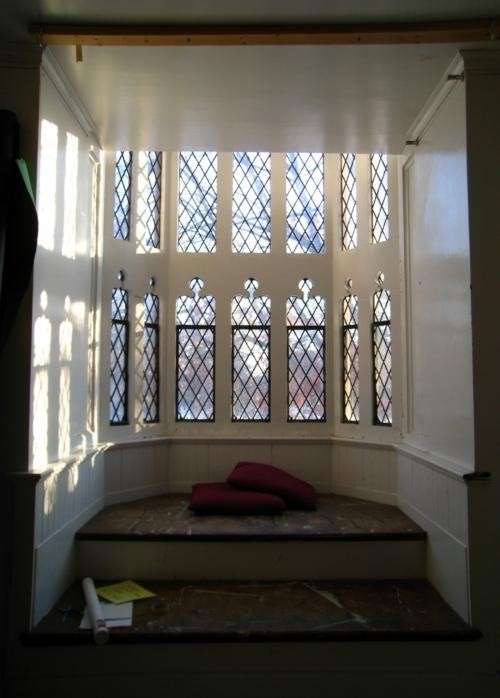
[76, 493, 427, 580]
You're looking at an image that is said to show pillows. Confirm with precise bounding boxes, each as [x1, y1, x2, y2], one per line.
[227, 461, 316, 502]
[189, 481, 286, 513]
